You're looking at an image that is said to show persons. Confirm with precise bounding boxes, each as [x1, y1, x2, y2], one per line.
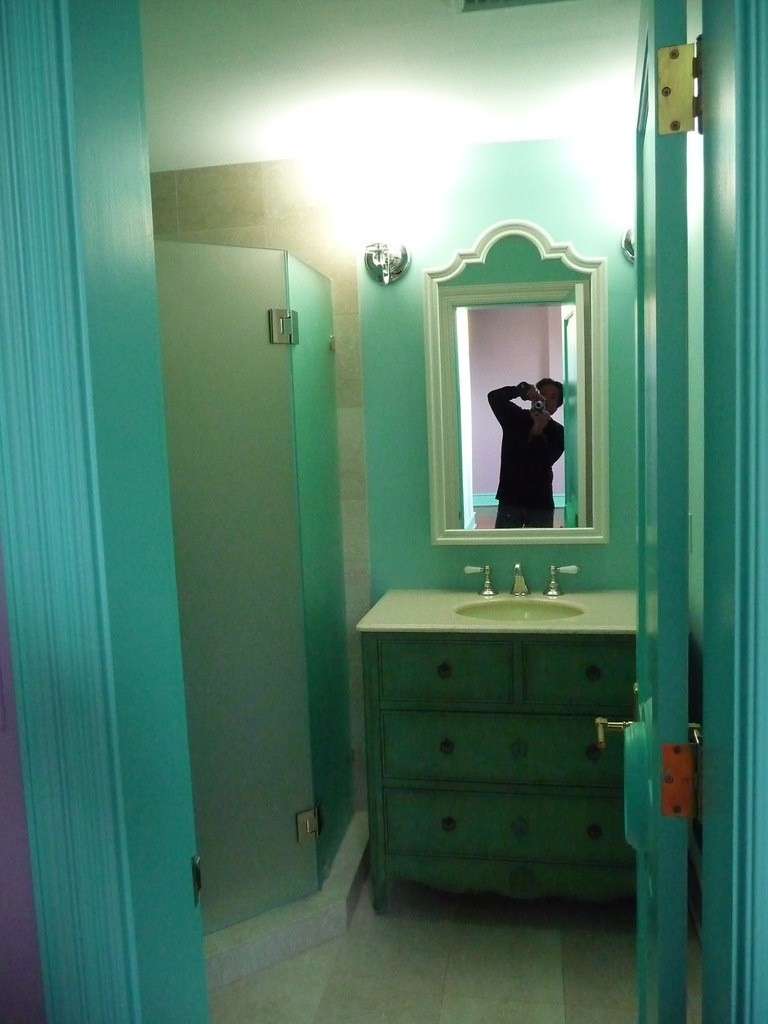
[487, 378, 565, 528]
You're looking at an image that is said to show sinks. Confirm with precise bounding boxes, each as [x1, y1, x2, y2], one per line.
[453, 591, 587, 623]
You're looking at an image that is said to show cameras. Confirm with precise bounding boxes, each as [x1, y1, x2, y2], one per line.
[532, 398, 545, 415]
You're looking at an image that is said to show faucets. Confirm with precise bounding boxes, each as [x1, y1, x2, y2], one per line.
[510, 562, 530, 596]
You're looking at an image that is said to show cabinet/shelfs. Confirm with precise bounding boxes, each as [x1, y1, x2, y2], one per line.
[361, 633, 636, 914]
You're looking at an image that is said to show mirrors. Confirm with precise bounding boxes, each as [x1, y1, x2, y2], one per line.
[420, 220, 610, 545]
